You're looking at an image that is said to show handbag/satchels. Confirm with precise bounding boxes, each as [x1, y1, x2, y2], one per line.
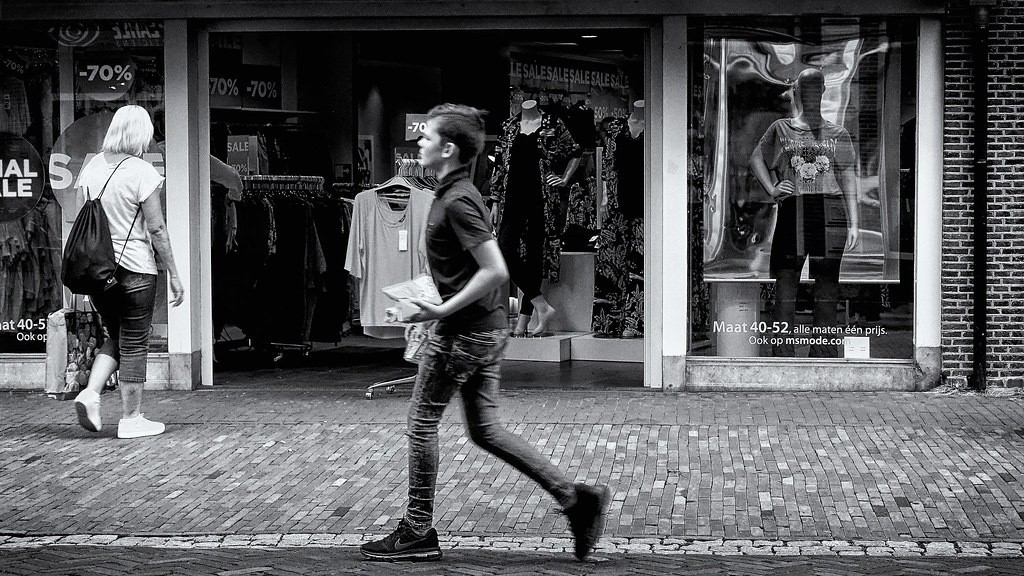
[46, 294, 118, 401]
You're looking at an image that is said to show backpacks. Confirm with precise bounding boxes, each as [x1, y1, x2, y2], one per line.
[61, 156, 141, 296]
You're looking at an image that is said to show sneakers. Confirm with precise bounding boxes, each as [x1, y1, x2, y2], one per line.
[553, 483, 611, 559]
[360, 514, 443, 562]
[118, 413, 165, 438]
[73, 389, 103, 431]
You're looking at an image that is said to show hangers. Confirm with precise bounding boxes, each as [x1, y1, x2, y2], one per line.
[374, 157, 439, 204]
[240, 174, 328, 198]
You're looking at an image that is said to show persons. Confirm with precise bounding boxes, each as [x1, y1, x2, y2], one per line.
[592, 100, 644, 339]
[485, 100, 583, 334]
[75, 104, 244, 438]
[360, 103, 611, 563]
[748, 69, 861, 358]
